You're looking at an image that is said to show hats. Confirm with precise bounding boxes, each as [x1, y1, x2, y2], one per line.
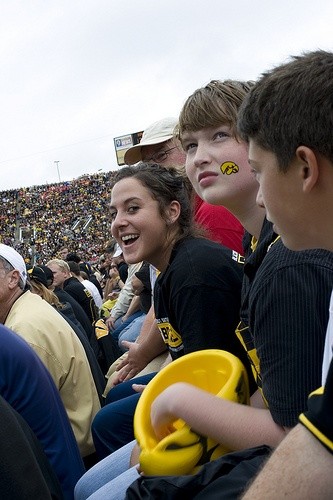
[28, 265, 54, 287]
[0, 242, 27, 285]
[78, 261, 92, 273]
[123, 118, 180, 165]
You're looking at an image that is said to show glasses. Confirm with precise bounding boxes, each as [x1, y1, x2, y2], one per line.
[142, 147, 178, 164]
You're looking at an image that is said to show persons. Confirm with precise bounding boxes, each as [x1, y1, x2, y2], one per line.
[0, 51, 333, 500]
[90, 162, 245, 459]
